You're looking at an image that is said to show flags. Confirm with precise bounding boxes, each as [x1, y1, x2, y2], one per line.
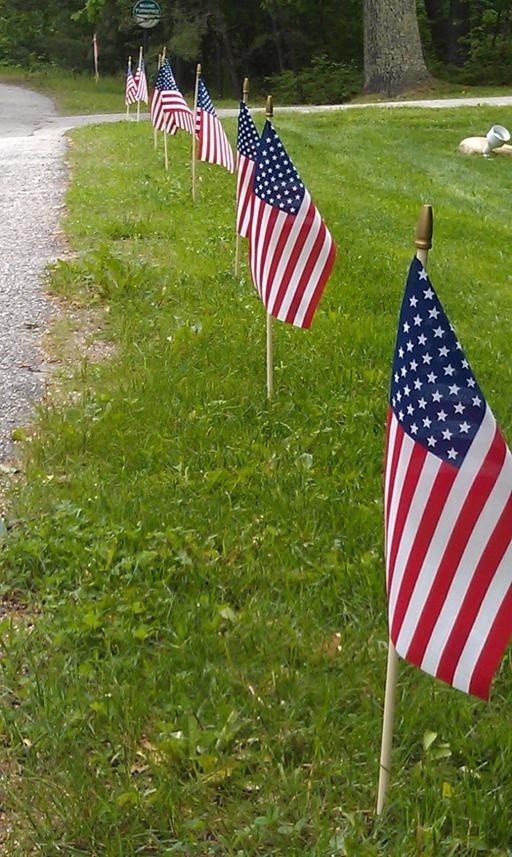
[235, 101, 260, 239]
[194, 76, 234, 174]
[384, 257, 512, 702]
[134, 57, 148, 106]
[150, 67, 178, 136]
[124, 61, 139, 106]
[161, 61, 195, 136]
[248, 118, 336, 329]
